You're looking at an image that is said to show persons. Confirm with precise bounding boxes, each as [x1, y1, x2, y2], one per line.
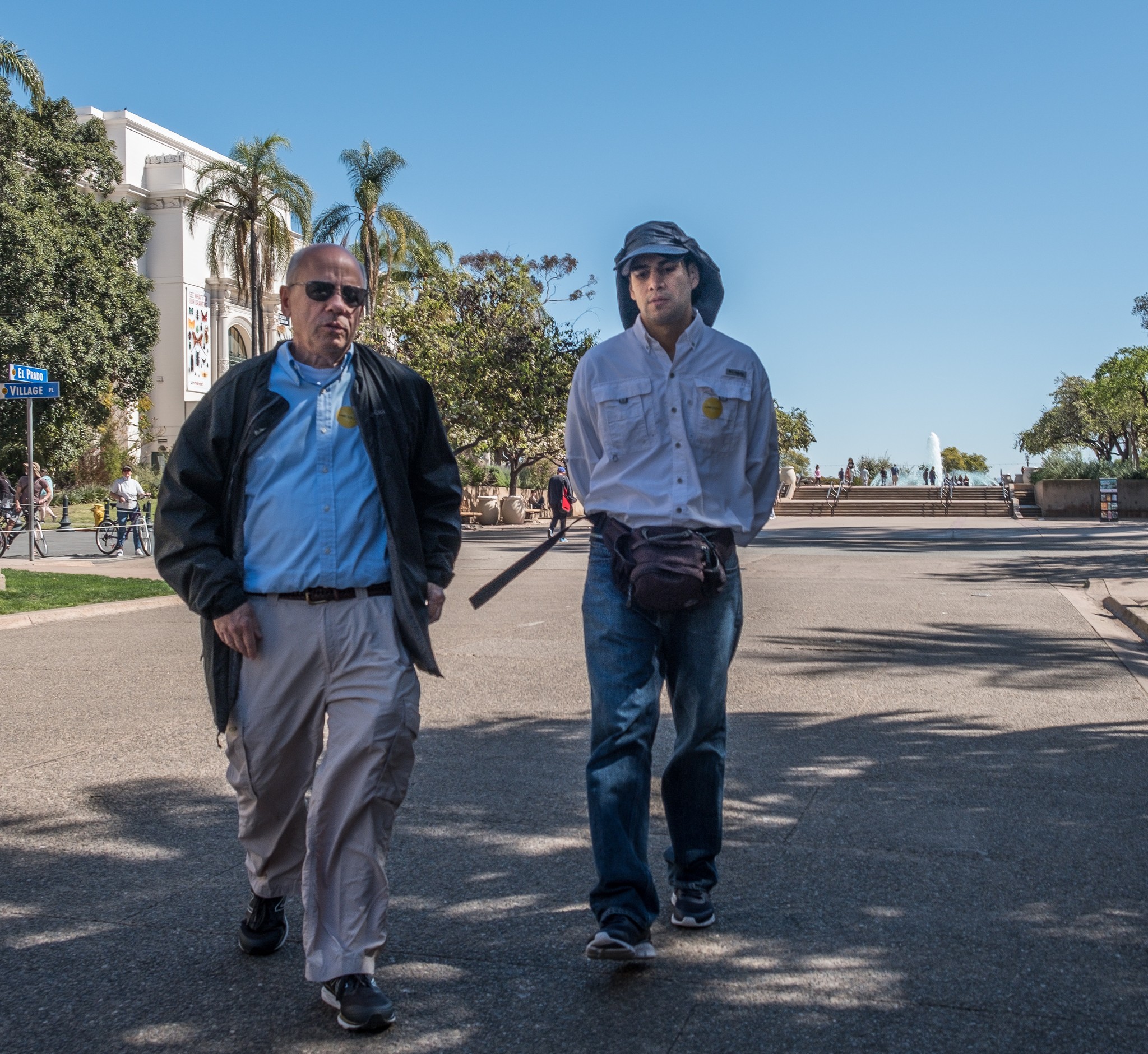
[110, 465, 152, 557]
[529, 490, 548, 510]
[562, 220, 783, 962]
[546, 467, 577, 543]
[0, 462, 51, 557]
[151, 241, 462, 1034]
[0, 471, 15, 523]
[794, 454, 1029, 489]
[39, 469, 57, 524]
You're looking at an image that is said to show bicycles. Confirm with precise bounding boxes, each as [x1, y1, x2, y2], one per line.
[96, 494, 153, 556]
[0, 501, 48, 558]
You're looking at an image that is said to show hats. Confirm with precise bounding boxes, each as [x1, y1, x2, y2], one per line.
[122, 466, 133, 473]
[22, 462, 42, 478]
[612, 221, 693, 273]
[558, 467, 566, 472]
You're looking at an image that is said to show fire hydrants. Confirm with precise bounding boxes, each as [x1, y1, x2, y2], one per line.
[90, 503, 105, 528]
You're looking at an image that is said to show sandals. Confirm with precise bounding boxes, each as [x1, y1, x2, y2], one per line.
[33, 550, 38, 556]
[6, 544, 10, 550]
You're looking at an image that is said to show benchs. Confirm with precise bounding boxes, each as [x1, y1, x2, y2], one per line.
[459, 500, 482, 524]
[525, 500, 542, 524]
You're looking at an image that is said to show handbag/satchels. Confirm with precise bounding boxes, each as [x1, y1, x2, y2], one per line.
[562, 476, 576, 512]
[610, 521, 728, 615]
[9, 485, 17, 493]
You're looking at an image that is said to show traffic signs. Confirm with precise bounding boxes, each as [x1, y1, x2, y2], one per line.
[0, 381, 60, 399]
[8, 363, 48, 383]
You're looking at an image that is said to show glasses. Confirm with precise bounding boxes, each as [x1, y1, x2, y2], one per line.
[123, 469, 132, 472]
[289, 279, 368, 306]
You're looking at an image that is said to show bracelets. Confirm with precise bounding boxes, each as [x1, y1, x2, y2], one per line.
[43, 497, 47, 500]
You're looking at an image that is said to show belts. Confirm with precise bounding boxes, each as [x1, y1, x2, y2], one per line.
[244, 584, 394, 600]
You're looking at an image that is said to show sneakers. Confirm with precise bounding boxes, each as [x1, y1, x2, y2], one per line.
[39, 520, 45, 523]
[559, 538, 568, 543]
[547, 529, 553, 539]
[671, 885, 715, 926]
[135, 548, 143, 555]
[52, 515, 58, 523]
[317, 972, 397, 1031]
[115, 549, 123, 556]
[236, 887, 289, 954]
[584, 914, 657, 960]
